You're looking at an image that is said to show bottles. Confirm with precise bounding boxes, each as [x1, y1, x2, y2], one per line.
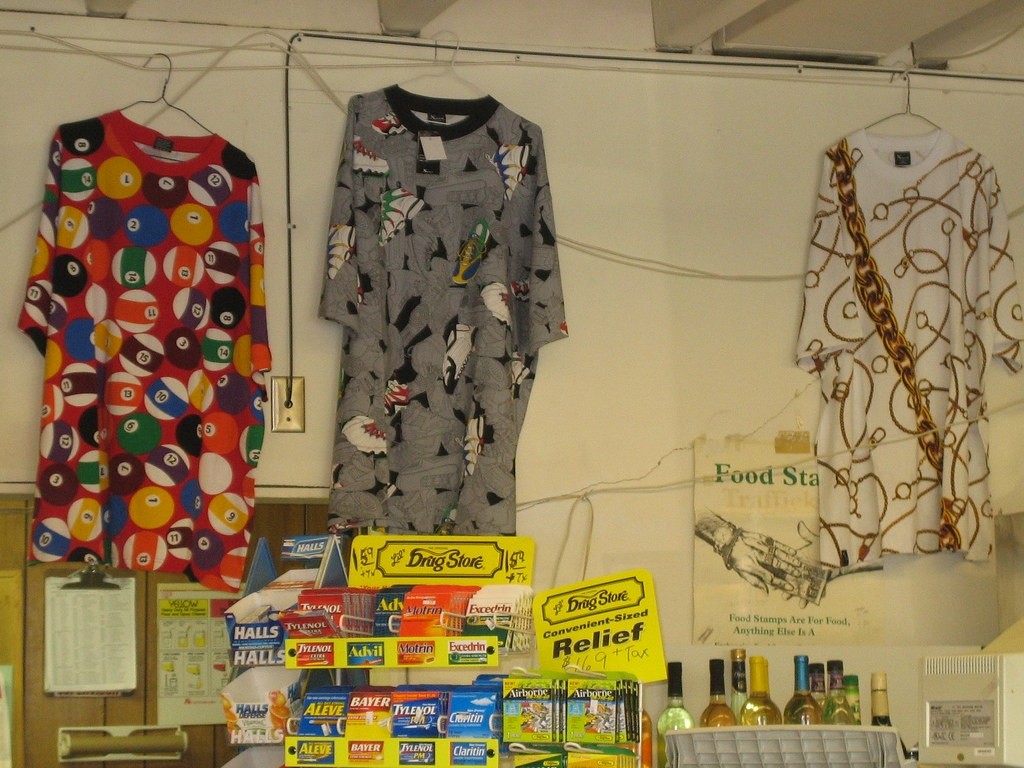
[741, 655, 775, 725]
[657, 661, 694, 768]
[784, 655, 825, 724]
[698, 659, 735, 727]
[641, 708, 653, 768]
[822, 660, 856, 726]
[763, 656, 783, 725]
[842, 674, 861, 727]
[870, 672, 907, 763]
[808, 662, 827, 708]
[731, 648, 749, 726]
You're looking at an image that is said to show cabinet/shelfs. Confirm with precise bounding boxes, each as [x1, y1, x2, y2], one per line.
[222, 533, 642, 768]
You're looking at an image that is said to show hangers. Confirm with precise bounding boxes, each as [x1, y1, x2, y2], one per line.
[119, 52, 217, 137]
[865, 72, 940, 132]
[396, 29, 486, 99]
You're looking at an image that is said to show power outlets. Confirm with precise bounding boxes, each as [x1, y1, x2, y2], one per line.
[272, 376, 306, 433]
